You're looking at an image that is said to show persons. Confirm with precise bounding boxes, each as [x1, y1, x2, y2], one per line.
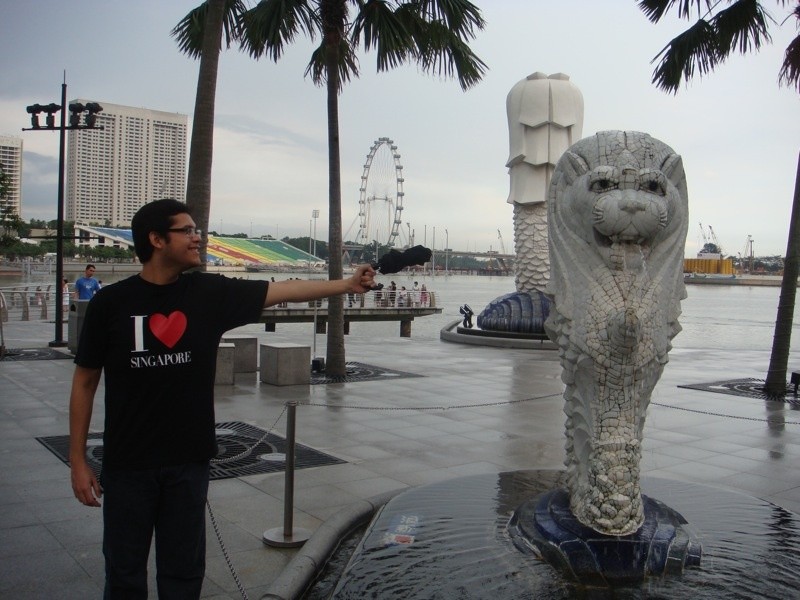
[66, 199, 377, 600]
[74, 264, 100, 300]
[376, 283, 384, 307]
[97, 280, 102, 289]
[347, 293, 356, 307]
[269, 275, 303, 308]
[398, 286, 407, 298]
[409, 281, 427, 308]
[62, 278, 69, 312]
[389, 281, 397, 307]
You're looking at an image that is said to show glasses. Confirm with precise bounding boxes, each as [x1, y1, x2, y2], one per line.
[165, 226, 202, 238]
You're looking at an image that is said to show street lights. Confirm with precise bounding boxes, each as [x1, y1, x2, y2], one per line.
[21, 69, 105, 348]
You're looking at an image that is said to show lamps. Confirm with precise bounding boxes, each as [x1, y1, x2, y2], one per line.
[460, 304, 472, 328]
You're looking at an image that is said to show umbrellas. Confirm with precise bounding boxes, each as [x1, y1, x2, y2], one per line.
[362, 244, 432, 290]
